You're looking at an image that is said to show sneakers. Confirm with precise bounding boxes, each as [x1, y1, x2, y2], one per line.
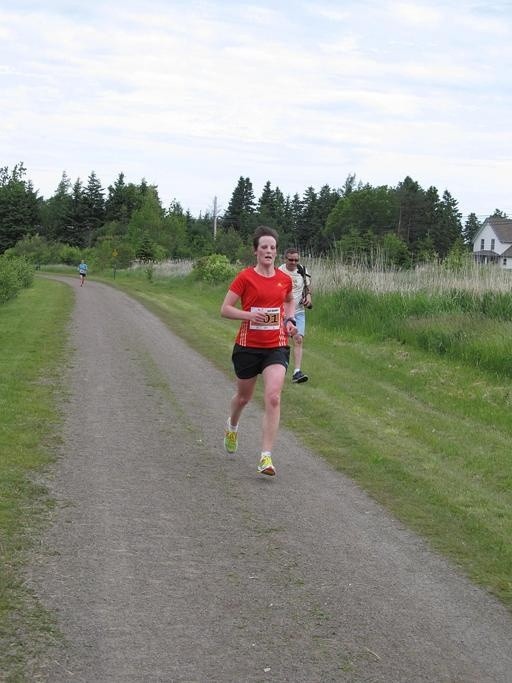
[256, 454, 277, 476]
[223, 417, 238, 456]
[291, 370, 309, 383]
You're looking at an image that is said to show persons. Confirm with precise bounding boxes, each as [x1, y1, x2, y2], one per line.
[219, 226, 297, 475]
[76, 260, 89, 284]
[278, 250, 315, 382]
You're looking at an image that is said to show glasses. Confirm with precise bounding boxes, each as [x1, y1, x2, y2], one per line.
[286, 255, 300, 263]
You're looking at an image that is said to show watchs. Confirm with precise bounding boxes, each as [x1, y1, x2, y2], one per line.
[285, 318, 297, 326]
